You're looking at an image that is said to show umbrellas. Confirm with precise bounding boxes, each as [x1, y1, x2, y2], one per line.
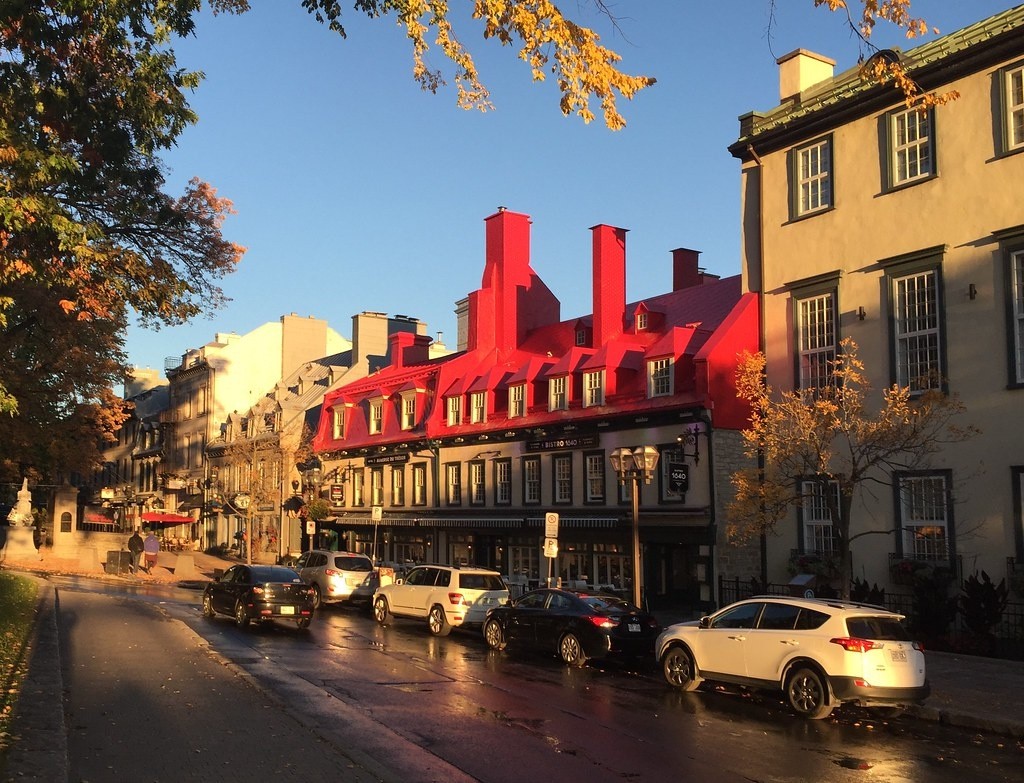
[128, 511, 194, 545]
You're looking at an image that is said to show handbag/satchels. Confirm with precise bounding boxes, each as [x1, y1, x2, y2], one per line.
[137, 545, 142, 552]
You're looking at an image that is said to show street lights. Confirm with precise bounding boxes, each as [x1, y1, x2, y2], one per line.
[609, 446, 660, 608]
[296, 456, 323, 551]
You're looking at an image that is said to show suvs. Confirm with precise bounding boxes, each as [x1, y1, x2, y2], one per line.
[287, 550, 380, 610]
[373, 564, 512, 636]
[655, 595, 930, 719]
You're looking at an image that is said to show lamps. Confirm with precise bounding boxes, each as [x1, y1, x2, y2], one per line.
[969, 284, 977, 300]
[859, 306, 866, 321]
[668, 424, 699, 464]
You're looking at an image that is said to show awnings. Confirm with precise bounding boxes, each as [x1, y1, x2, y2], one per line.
[524, 513, 623, 528]
[418, 516, 525, 530]
[336, 516, 416, 527]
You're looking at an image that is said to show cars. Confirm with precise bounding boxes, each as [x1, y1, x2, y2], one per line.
[483, 588, 661, 667]
[203, 564, 315, 629]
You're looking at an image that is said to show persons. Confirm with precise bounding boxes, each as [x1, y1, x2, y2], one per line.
[144, 531, 160, 574]
[128, 532, 144, 574]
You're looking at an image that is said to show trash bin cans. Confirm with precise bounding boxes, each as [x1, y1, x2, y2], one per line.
[106, 550, 131, 574]
[528, 579, 540, 591]
